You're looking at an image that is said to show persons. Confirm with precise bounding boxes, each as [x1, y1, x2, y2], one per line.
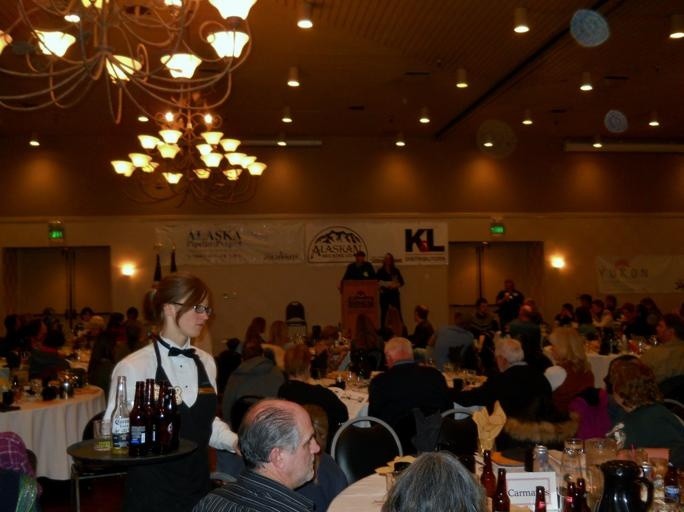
[0, 251, 683, 512]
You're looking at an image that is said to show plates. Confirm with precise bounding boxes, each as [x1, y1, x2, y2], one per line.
[374, 459, 410, 475]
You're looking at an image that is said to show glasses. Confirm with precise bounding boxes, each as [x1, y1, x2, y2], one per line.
[163, 300, 212, 316]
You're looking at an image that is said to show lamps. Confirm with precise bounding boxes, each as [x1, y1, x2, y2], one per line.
[110, 83, 268, 210]
[0, 1, 259, 127]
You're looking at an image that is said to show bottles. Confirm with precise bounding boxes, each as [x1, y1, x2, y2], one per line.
[561, 477, 590, 512]
[480, 450, 496, 511]
[111, 375, 179, 457]
[535, 487, 547, 510]
[664, 446, 681, 503]
[491, 467, 510, 510]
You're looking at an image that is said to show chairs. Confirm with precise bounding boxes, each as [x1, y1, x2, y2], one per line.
[0, 340, 682, 510]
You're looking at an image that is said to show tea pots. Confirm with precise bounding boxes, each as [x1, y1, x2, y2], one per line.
[593, 459, 653, 511]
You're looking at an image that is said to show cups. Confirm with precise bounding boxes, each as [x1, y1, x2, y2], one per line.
[330, 362, 369, 391]
[385, 472, 398, 492]
[427, 358, 475, 388]
[92, 420, 113, 453]
[2, 370, 76, 406]
[560, 437, 646, 497]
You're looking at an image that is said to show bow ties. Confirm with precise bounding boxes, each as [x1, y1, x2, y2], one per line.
[167, 347, 195, 358]
